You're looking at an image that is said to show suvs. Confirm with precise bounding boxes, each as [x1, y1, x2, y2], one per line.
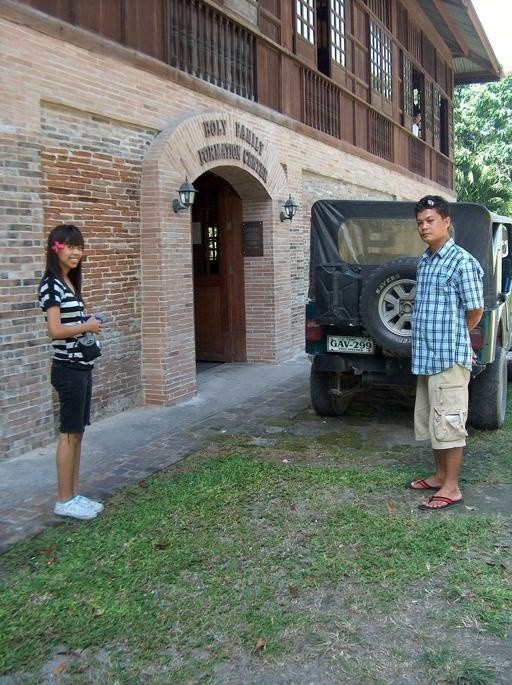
[306, 199, 512, 429]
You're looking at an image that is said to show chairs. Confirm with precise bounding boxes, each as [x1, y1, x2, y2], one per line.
[356, 224, 406, 263]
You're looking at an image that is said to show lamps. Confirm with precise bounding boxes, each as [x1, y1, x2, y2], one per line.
[281, 192, 297, 222]
[172, 174, 197, 213]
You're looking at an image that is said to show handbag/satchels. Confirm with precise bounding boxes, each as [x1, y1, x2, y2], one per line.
[78, 335, 104, 362]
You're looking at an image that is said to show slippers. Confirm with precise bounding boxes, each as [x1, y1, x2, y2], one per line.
[406, 479, 434, 490]
[417, 494, 462, 511]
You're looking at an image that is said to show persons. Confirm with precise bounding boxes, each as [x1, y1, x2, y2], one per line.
[37, 223, 104, 522]
[404, 195, 485, 512]
[412, 105, 422, 138]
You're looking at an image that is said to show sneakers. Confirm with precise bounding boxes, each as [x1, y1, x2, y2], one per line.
[53, 496, 104, 522]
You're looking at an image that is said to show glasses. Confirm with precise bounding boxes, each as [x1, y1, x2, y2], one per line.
[416, 198, 440, 207]
